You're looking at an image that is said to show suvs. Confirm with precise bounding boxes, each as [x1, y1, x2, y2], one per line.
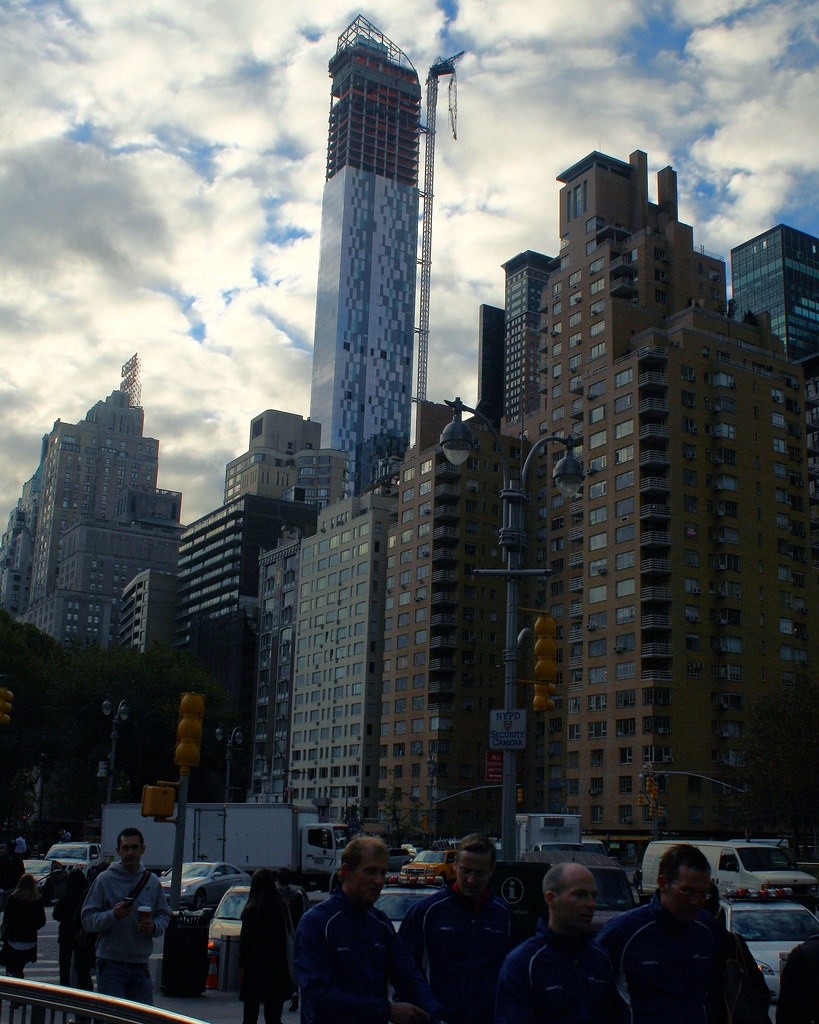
[39, 841, 103, 888]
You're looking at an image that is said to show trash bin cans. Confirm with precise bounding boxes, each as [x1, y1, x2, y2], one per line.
[161, 914, 212, 998]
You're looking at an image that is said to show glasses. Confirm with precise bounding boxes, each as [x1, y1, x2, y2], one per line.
[667, 879, 710, 904]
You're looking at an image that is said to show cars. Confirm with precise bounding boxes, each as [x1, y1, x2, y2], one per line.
[22, 859, 68, 908]
[397, 839, 459, 888]
[715, 888, 819, 1003]
[156, 861, 253, 912]
[385, 845, 424, 872]
[208, 886, 251, 940]
[373, 886, 440, 934]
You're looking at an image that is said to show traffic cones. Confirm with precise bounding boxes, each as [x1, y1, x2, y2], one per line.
[204, 955, 220, 989]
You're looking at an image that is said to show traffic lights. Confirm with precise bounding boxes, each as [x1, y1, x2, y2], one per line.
[533, 681, 556, 712]
[645, 777, 654, 793]
[637, 795, 644, 806]
[657, 806, 664, 817]
[650, 804, 656, 817]
[650, 785, 658, 800]
[0, 687, 14, 725]
[517, 788, 524, 803]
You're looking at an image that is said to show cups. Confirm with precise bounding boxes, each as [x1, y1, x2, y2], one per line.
[138, 906, 152, 924]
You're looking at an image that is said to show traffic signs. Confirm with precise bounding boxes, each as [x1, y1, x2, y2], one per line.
[486, 750, 503, 782]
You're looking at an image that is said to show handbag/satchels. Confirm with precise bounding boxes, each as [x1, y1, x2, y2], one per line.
[285, 905, 297, 984]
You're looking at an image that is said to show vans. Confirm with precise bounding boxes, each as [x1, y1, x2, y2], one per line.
[641, 839, 819, 916]
[519, 852, 638, 937]
[581, 838, 618, 861]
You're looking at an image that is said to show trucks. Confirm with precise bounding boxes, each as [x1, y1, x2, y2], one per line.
[515, 812, 587, 862]
[101, 802, 351, 893]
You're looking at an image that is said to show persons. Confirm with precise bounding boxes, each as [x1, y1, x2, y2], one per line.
[494, 862, 613, 1024]
[293, 835, 448, 1024]
[277, 867, 303, 932]
[776, 934, 819, 1024]
[594, 845, 773, 1024]
[81, 828, 170, 1006]
[238, 869, 293, 1024]
[0, 831, 108, 1024]
[392, 832, 515, 1024]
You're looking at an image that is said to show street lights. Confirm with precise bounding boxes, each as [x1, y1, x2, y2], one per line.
[101, 695, 129, 805]
[439, 396, 586, 863]
[215, 723, 246, 803]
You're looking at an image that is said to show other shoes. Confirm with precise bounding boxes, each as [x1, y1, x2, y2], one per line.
[289, 995, 298, 1011]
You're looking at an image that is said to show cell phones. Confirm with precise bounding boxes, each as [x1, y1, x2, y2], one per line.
[123, 898, 134, 909]
